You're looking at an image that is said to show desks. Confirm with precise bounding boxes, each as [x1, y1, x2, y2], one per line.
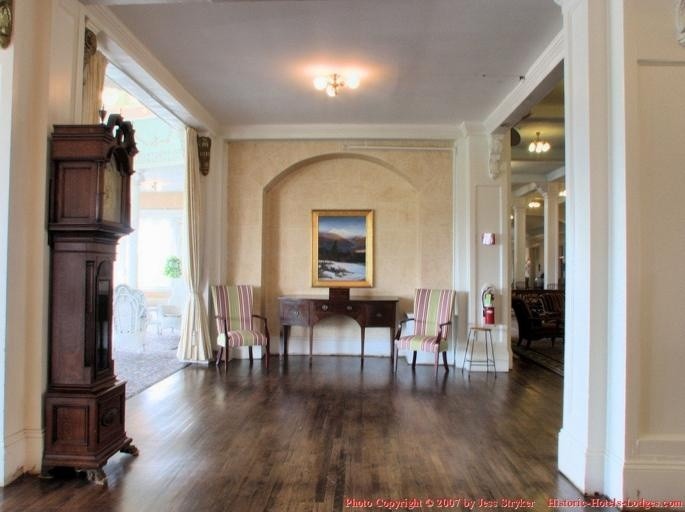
[278, 296, 400, 369]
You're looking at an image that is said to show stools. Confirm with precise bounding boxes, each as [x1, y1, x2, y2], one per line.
[462, 327, 496, 379]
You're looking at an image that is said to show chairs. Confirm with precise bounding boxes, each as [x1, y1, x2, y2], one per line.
[209, 284, 270, 375]
[112, 279, 185, 351]
[44, 105, 139, 486]
[393, 288, 456, 379]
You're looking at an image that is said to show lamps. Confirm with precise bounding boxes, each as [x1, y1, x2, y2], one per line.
[528, 132, 551, 153]
[314, 71, 359, 96]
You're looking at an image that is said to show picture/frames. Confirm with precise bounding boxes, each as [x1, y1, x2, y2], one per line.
[311, 208, 374, 288]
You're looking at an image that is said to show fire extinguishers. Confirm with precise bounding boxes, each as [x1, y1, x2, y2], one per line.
[482, 286, 494, 325]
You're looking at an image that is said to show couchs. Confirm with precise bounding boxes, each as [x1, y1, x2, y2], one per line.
[512, 289, 564, 351]
